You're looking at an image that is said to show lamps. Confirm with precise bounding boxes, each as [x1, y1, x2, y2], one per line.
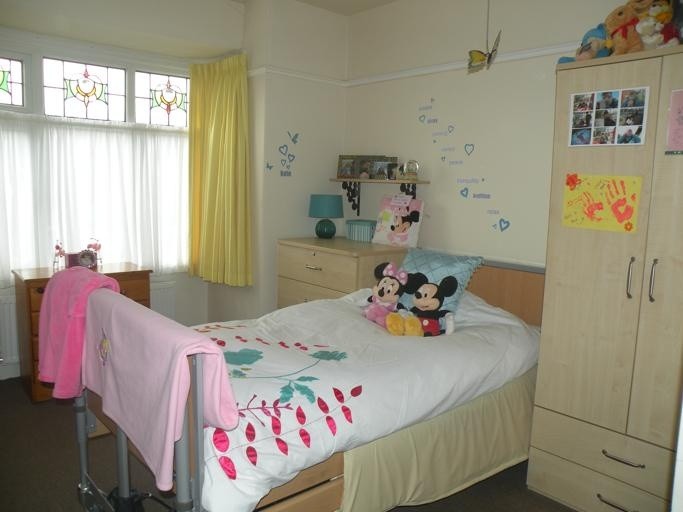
[308, 194, 344, 239]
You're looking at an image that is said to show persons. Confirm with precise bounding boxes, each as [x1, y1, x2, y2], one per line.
[341, 158, 396, 181]
[573, 90, 645, 145]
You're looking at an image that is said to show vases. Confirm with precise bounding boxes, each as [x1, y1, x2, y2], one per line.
[53, 257, 59, 272]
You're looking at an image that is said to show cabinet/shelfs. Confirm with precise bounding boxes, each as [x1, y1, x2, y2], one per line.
[276, 237, 406, 311]
[525, 46, 683, 512]
[14, 270, 155, 404]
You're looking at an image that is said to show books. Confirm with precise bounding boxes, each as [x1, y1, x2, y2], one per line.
[372, 195, 424, 248]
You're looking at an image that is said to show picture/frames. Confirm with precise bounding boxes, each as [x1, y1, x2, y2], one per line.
[338, 155, 397, 179]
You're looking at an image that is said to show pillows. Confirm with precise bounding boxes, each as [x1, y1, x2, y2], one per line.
[394, 248, 484, 329]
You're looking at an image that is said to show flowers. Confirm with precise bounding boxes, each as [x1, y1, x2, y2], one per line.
[54, 240, 65, 259]
[87, 238, 101, 253]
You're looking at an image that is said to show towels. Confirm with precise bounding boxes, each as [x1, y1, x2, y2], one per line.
[37, 266, 120, 400]
[81, 287, 239, 492]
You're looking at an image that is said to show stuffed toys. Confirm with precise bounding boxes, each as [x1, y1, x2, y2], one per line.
[383, 269, 459, 338]
[553, 0, 683, 65]
[360, 262, 417, 330]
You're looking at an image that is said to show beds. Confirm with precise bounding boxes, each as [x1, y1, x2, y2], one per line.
[36, 248, 547, 512]
